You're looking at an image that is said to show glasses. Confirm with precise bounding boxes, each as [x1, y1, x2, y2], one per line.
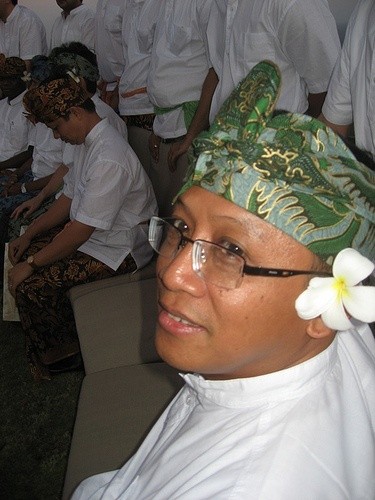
[149, 216, 333, 289]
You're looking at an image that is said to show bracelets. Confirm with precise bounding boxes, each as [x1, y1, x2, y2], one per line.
[21, 182, 27, 193]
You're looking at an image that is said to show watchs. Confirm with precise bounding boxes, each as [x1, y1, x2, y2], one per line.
[27, 255, 39, 271]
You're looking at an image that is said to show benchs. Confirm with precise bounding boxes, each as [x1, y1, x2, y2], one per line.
[63, 126, 192, 499]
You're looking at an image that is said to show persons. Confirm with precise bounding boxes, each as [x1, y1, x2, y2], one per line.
[8, 74, 159, 386]
[318, 0, 375, 171]
[94, 0, 212, 159]
[71, 59, 375, 500]
[0, 0, 47, 72]
[0, 41, 128, 243]
[0, 53, 66, 198]
[167, 0, 342, 171]
[47, 0, 95, 55]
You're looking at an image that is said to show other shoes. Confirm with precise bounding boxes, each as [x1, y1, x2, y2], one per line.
[48, 354, 82, 371]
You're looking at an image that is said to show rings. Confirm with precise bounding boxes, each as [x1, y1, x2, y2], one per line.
[154, 144, 158, 148]
